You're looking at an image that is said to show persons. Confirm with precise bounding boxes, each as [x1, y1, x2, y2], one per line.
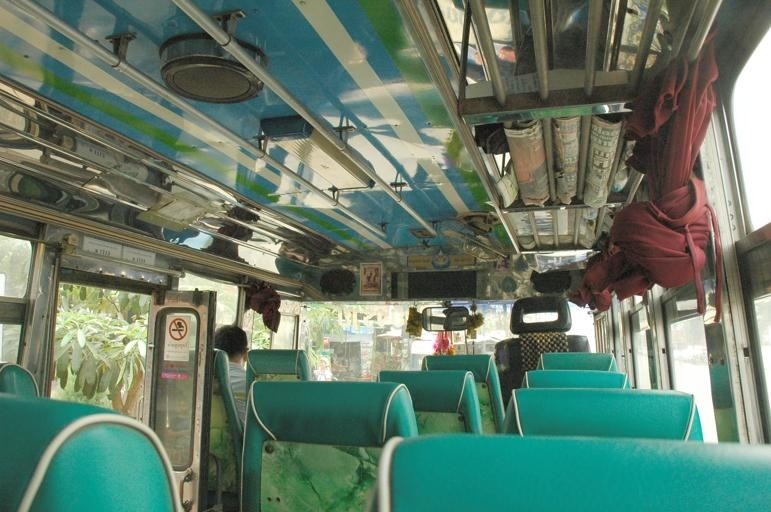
[215, 324, 250, 440]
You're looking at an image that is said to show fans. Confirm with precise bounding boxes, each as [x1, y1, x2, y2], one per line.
[440, 224, 508, 262]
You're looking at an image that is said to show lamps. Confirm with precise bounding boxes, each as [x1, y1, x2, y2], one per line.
[260, 114, 376, 193]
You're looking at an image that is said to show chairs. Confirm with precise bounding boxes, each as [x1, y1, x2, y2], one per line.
[1, 360, 185, 509]
[207, 296, 768, 510]
